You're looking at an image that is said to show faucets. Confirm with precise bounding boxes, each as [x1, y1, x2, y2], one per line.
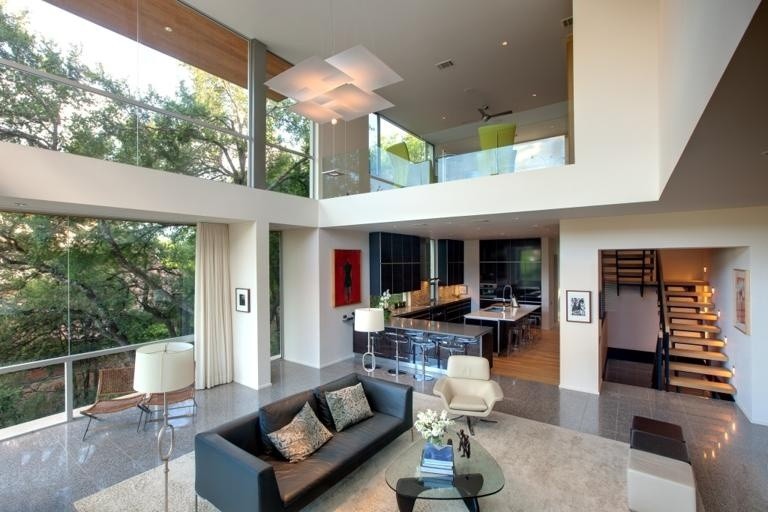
[503, 284, 513, 302]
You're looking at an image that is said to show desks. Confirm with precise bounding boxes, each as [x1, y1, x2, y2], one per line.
[463, 302, 540, 356]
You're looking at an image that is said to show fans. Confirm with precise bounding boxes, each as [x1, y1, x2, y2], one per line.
[473, 105, 514, 124]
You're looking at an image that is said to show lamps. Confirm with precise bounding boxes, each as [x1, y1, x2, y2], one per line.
[133, 342, 195, 511]
[354, 308, 385, 377]
[264, 1, 404, 125]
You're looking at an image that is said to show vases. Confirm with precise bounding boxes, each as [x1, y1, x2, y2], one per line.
[429, 436, 442, 444]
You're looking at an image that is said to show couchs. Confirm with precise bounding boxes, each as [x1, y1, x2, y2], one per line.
[195, 373, 414, 511]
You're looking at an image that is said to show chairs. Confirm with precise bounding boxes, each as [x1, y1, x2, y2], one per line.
[79, 366, 145, 439]
[433, 355, 503, 436]
[386, 143, 434, 187]
[145, 361, 196, 429]
[476, 124, 517, 175]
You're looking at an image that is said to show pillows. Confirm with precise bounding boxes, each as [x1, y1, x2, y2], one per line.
[266, 400, 333, 464]
[324, 383, 373, 432]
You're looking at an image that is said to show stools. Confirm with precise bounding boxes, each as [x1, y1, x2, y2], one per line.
[628, 415, 697, 512]
[370, 330, 478, 383]
[502, 323, 520, 356]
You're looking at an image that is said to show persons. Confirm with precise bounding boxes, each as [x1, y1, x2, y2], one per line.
[344, 256, 353, 303]
[456, 429, 465, 451]
[461, 434, 470, 458]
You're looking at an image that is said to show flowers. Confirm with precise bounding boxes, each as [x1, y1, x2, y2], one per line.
[414, 409, 456, 440]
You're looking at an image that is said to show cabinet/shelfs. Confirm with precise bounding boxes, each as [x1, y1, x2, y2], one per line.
[369, 231, 471, 327]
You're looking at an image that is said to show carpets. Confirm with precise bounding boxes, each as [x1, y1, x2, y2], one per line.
[73, 390, 632, 512]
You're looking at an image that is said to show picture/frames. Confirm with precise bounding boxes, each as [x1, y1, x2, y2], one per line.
[235, 288, 250, 312]
[566, 290, 591, 324]
[331, 249, 363, 309]
[732, 269, 750, 335]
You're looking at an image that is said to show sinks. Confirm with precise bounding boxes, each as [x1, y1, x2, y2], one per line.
[484, 307, 502, 312]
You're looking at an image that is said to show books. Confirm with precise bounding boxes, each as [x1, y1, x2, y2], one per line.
[418, 473, 454, 490]
[419, 442, 455, 476]
